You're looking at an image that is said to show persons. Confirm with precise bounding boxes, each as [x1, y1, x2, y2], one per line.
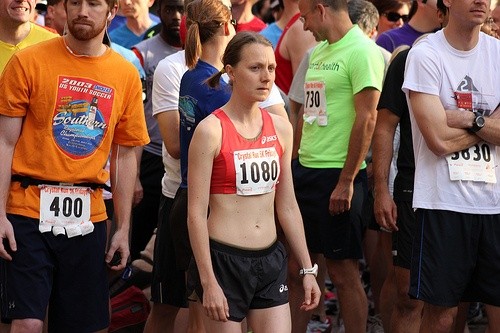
[0, 0, 500, 333]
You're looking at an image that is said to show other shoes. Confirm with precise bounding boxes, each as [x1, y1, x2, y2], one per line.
[304, 317, 332, 333]
[325, 297, 338, 314]
[367, 315, 385, 333]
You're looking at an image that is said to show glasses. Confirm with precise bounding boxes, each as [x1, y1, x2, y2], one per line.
[218, 19, 236, 27]
[381, 11, 409, 23]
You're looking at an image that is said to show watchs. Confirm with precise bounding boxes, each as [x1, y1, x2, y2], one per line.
[469, 112, 485, 133]
[299, 262, 318, 278]
